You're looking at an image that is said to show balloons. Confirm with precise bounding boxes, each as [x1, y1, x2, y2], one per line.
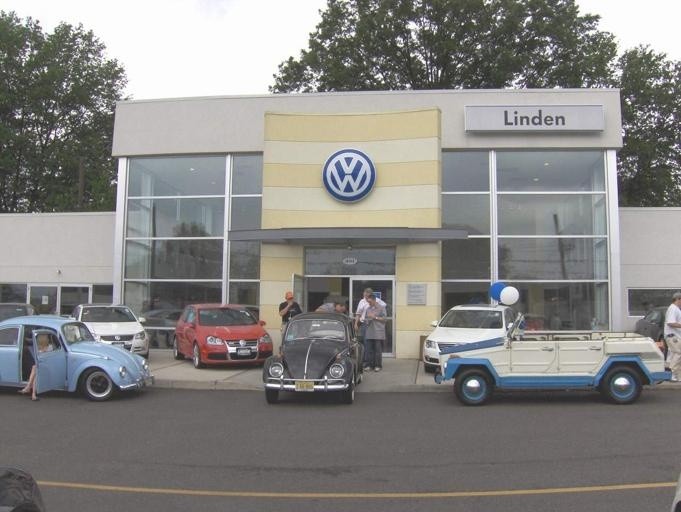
[499, 286, 519, 305]
[488, 282, 506, 300]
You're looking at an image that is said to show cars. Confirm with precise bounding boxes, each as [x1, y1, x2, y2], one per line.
[0, 313, 154, 402]
[71, 302, 150, 359]
[172, 303, 274, 369]
[434, 311, 673, 405]
[421, 302, 517, 373]
[262, 311, 365, 404]
[635, 306, 668, 342]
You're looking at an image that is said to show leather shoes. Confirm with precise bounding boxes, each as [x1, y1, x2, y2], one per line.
[18, 389, 37, 401]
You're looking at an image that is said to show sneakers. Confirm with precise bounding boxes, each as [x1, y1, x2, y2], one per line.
[363, 365, 381, 373]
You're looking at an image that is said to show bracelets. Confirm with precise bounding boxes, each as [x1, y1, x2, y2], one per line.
[375, 317, 378, 320]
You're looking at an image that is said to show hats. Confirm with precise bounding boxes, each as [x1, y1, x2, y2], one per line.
[286, 292, 293, 299]
[673, 292, 681, 300]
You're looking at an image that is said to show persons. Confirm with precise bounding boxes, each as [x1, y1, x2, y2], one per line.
[354, 288, 387, 367]
[314, 302, 347, 314]
[279, 292, 302, 351]
[18, 334, 53, 401]
[364, 295, 386, 372]
[664, 292, 681, 382]
